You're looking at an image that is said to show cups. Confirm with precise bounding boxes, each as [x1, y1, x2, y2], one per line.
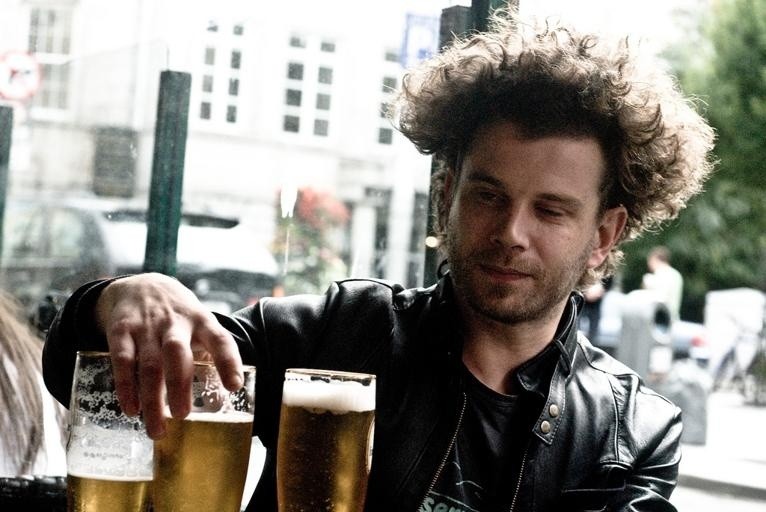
[276, 368, 378, 512]
[150, 364, 257, 512]
[66, 350, 156, 511]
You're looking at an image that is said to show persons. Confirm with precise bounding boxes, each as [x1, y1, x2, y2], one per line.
[41, 10, 716, 512]
[34, 292, 56, 327]
[576, 268, 614, 344]
[640, 245, 682, 336]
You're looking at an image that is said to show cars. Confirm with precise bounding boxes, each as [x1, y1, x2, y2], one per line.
[3, 197, 284, 332]
